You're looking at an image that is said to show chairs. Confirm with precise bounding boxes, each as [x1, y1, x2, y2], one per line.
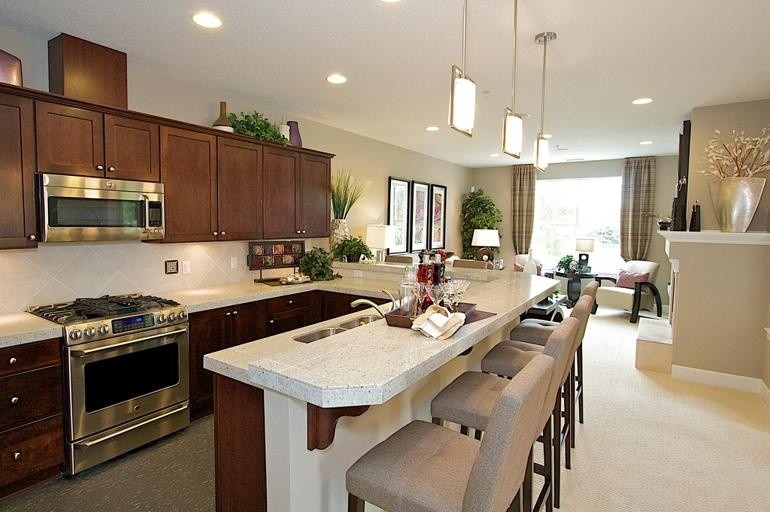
[346, 354, 555, 512]
[507, 280, 600, 448]
[385, 255, 413, 264]
[514, 252, 543, 275]
[592, 259, 664, 324]
[430, 317, 580, 512]
[453, 259, 488, 269]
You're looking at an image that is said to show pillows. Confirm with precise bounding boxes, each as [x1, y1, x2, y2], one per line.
[614, 268, 651, 291]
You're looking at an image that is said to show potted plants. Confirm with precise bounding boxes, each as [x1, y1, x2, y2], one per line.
[328, 168, 367, 257]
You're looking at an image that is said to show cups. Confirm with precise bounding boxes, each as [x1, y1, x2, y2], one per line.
[493, 258, 504, 270]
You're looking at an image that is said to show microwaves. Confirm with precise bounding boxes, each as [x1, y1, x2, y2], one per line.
[35, 171, 166, 245]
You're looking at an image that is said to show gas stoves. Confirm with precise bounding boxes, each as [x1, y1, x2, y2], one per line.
[24, 291, 190, 346]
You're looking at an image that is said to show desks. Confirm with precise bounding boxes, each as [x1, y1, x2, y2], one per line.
[554, 271, 599, 307]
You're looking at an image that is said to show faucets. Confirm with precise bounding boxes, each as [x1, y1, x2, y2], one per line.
[350, 299, 386, 319]
[382, 290, 398, 311]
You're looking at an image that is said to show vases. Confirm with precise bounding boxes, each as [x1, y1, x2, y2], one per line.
[287, 120, 303, 146]
[212, 100, 235, 132]
[706, 176, 766, 233]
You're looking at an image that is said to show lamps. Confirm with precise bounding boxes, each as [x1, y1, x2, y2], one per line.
[532, 31, 557, 174]
[365, 223, 396, 262]
[450, 0, 478, 137]
[502, 0, 526, 161]
[575, 238, 595, 267]
[471, 228, 500, 261]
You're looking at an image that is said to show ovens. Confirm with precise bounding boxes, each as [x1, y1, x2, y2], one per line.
[64, 323, 192, 477]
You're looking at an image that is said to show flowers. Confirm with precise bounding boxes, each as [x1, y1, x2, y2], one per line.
[696, 125, 769, 180]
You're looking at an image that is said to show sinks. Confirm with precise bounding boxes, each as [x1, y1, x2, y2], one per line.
[294, 329, 346, 343]
[340, 316, 381, 330]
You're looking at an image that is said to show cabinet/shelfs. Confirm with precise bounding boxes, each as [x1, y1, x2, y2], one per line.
[320, 288, 393, 323]
[0, 79, 42, 250]
[142, 114, 263, 244]
[33, 89, 160, 183]
[189, 297, 268, 425]
[0, 330, 71, 500]
[263, 140, 336, 240]
[269, 289, 319, 337]
[209, 370, 268, 512]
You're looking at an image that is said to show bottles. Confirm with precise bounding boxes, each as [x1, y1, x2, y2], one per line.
[398, 253, 470, 316]
[689, 198, 701, 232]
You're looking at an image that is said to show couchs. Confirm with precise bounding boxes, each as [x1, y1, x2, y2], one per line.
[404, 252, 459, 265]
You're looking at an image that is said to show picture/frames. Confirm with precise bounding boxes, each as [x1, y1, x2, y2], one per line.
[430, 183, 447, 250]
[386, 175, 411, 255]
[411, 179, 430, 253]
